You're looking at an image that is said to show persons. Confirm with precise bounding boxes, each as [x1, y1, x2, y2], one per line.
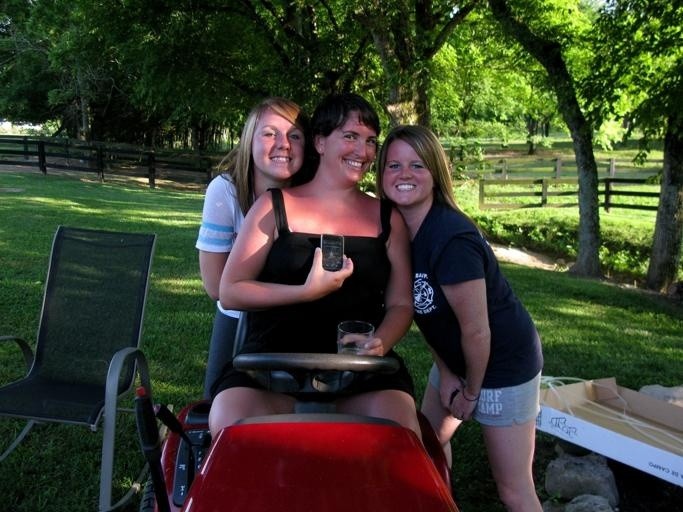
[196, 95, 307, 407]
[208, 93, 423, 442]
[376, 124, 541, 512]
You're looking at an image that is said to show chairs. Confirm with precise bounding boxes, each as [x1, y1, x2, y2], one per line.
[0, 221, 169, 510]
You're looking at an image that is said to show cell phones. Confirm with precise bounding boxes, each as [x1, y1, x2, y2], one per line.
[321, 233, 344, 271]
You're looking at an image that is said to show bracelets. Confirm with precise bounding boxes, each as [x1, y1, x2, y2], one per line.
[462, 386, 479, 402]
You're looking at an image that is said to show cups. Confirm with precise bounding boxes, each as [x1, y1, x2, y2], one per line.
[336, 318, 375, 356]
